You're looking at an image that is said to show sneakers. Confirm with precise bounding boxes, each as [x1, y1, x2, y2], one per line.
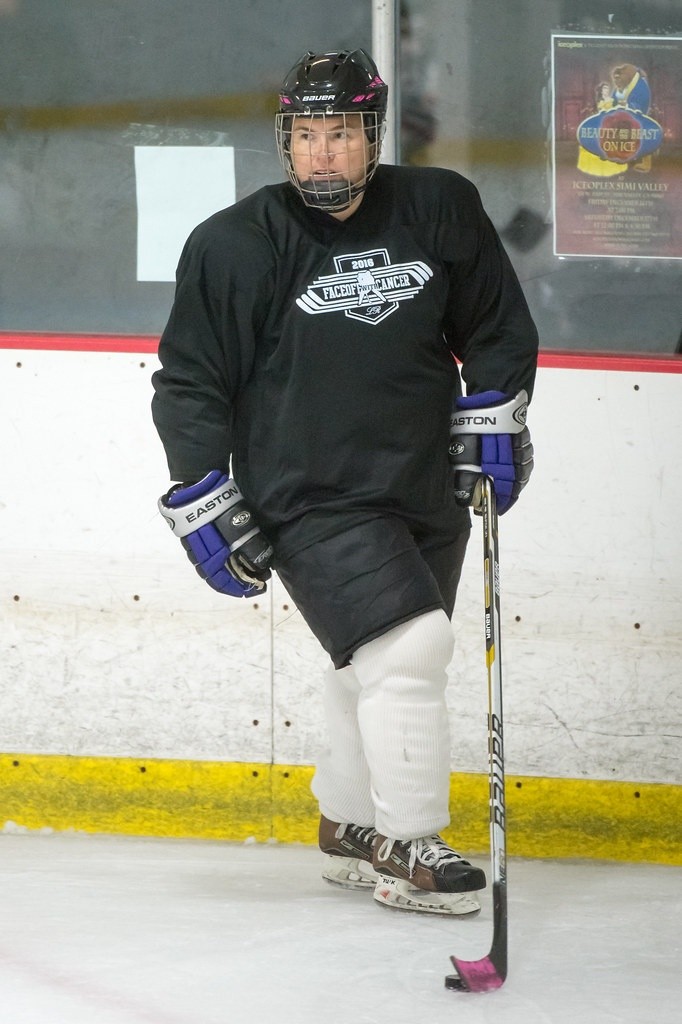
[318, 814, 487, 918]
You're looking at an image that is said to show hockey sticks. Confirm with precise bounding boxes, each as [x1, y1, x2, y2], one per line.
[450, 476, 508, 994]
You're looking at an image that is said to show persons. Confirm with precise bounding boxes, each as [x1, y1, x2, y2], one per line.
[150, 48, 540, 918]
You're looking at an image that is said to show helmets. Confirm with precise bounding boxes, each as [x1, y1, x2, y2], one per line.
[277, 49, 390, 145]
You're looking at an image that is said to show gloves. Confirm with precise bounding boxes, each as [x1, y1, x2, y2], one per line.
[451, 386, 535, 519]
[156, 467, 275, 599]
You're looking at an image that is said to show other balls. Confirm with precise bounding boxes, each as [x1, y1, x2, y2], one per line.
[445, 973, 463, 991]
